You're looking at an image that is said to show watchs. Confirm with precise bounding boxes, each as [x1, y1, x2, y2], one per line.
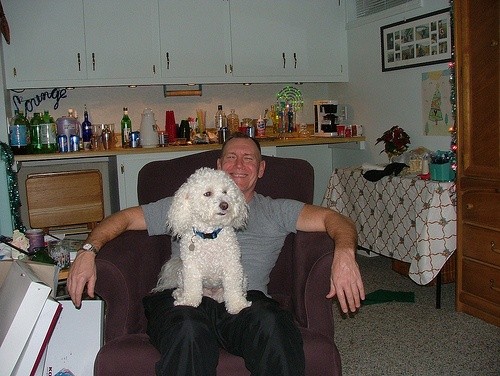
[77, 243, 98, 255]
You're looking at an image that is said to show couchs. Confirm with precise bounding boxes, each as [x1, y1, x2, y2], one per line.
[65, 148, 342, 376]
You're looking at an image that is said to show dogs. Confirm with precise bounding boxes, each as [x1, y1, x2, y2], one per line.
[148, 167, 253, 314]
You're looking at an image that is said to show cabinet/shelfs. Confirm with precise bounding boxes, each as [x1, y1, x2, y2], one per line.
[0, 0, 350, 89]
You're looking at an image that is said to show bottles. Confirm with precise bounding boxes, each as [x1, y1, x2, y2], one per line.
[26, 229, 45, 252]
[214, 102, 296, 137]
[8, 110, 57, 153]
[121, 107, 131, 148]
[81, 112, 92, 151]
[139, 108, 159, 149]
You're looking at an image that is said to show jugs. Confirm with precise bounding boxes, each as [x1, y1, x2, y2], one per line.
[55, 116, 82, 151]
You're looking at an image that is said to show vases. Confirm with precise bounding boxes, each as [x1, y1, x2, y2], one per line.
[385, 150, 402, 163]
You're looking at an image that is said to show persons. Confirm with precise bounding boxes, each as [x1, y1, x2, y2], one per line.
[67, 131, 364, 376]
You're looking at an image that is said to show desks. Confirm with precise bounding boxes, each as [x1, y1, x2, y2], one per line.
[320, 161, 457, 309]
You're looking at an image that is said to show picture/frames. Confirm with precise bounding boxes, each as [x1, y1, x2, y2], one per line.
[380, 6, 454, 72]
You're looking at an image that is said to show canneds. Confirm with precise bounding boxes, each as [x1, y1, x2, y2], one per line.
[69, 134, 80, 152]
[58, 134, 68, 152]
[247, 125, 255, 138]
[129, 132, 138, 148]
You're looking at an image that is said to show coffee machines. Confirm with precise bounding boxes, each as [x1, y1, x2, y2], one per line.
[311, 99, 340, 137]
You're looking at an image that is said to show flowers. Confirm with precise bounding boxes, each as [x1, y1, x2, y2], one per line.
[374, 125, 411, 158]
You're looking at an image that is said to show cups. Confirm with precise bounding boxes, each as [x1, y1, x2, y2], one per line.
[48, 239, 71, 270]
[165, 110, 205, 143]
[337, 125, 345, 137]
[91, 123, 117, 151]
[218, 130, 229, 143]
